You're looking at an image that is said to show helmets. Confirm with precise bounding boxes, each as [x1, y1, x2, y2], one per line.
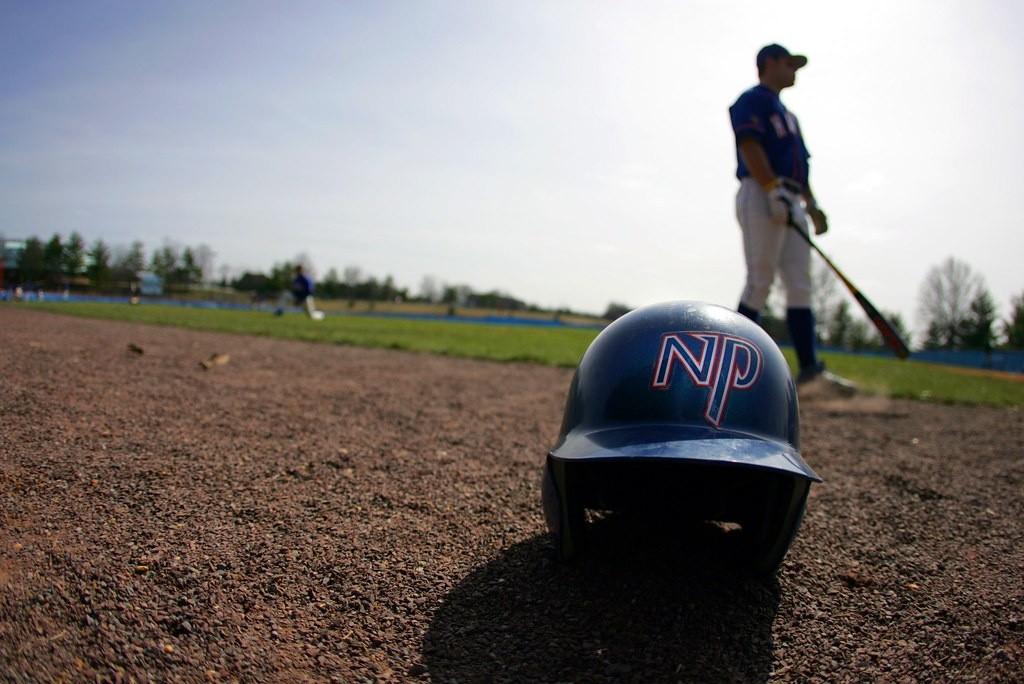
[546, 301, 822, 556]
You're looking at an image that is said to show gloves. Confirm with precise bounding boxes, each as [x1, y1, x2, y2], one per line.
[768, 189, 791, 224]
[809, 207, 827, 233]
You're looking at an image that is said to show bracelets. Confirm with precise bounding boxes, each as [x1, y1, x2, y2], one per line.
[763, 177, 783, 193]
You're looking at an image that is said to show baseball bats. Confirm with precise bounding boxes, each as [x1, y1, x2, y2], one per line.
[788, 217, 913, 363]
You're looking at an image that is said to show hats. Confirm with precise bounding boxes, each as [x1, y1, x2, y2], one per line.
[757, 44, 807, 70]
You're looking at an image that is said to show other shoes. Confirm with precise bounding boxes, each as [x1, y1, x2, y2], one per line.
[799, 360, 823, 381]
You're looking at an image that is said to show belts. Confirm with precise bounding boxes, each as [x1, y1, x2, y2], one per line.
[782, 181, 802, 193]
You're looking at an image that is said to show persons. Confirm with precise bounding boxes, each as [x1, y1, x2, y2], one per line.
[274, 265, 326, 319]
[728, 43, 857, 400]
